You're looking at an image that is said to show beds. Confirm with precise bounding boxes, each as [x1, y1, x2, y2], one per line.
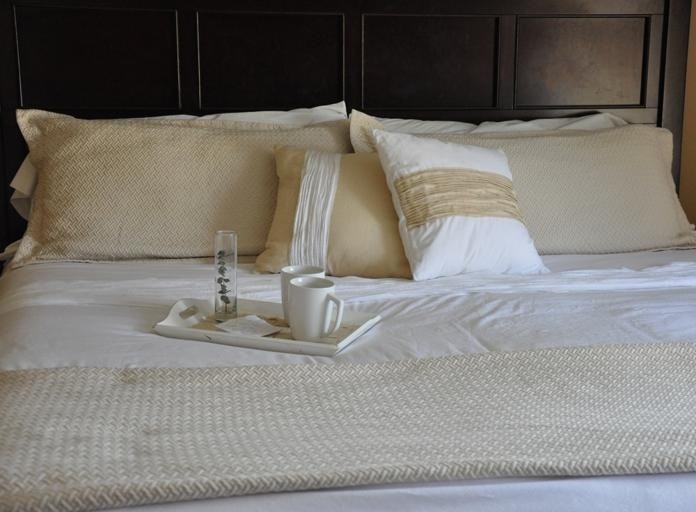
[0, 0, 696, 512]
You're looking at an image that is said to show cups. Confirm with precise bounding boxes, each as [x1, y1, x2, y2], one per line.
[212, 228, 240, 324]
[279, 263, 326, 326]
[280, 276, 345, 342]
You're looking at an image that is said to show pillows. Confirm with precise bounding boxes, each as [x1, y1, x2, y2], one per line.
[380, 121, 695, 255]
[251, 143, 419, 279]
[369, 126, 553, 281]
[347, 106, 628, 156]
[8, 107, 346, 274]
[11, 101, 352, 231]
